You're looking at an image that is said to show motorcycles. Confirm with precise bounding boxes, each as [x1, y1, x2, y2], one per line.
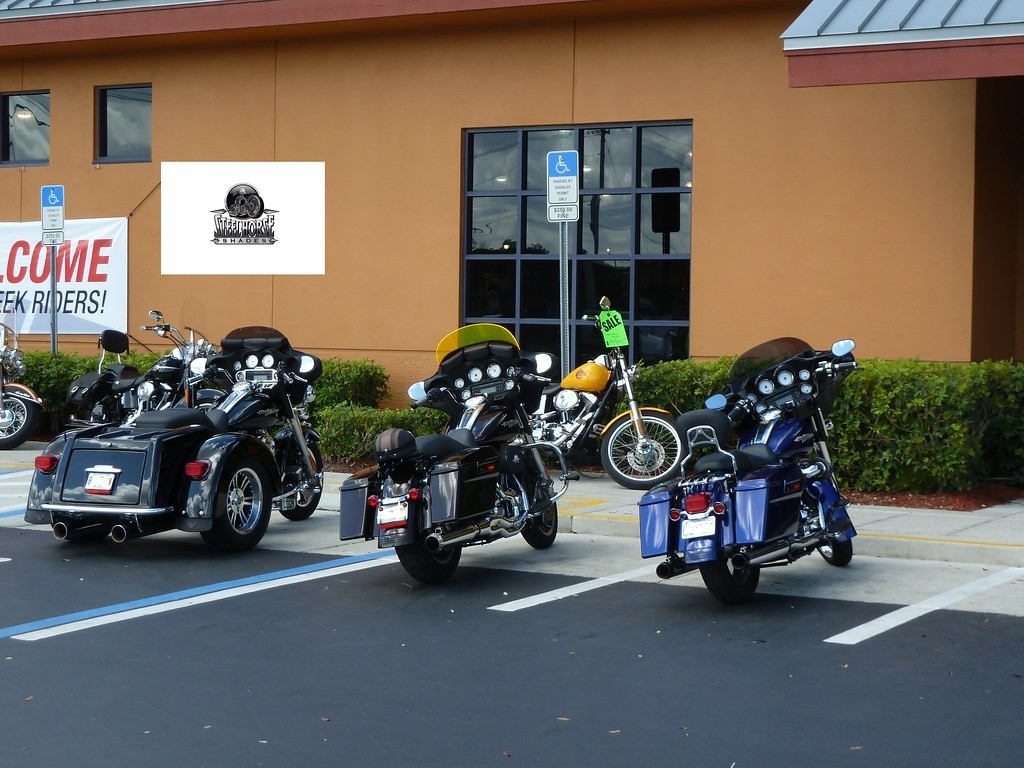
[339, 321, 581, 586]
[0, 308, 46, 451]
[638, 336, 861, 606]
[523, 298, 691, 490]
[61, 310, 222, 429]
[25, 325, 325, 551]
